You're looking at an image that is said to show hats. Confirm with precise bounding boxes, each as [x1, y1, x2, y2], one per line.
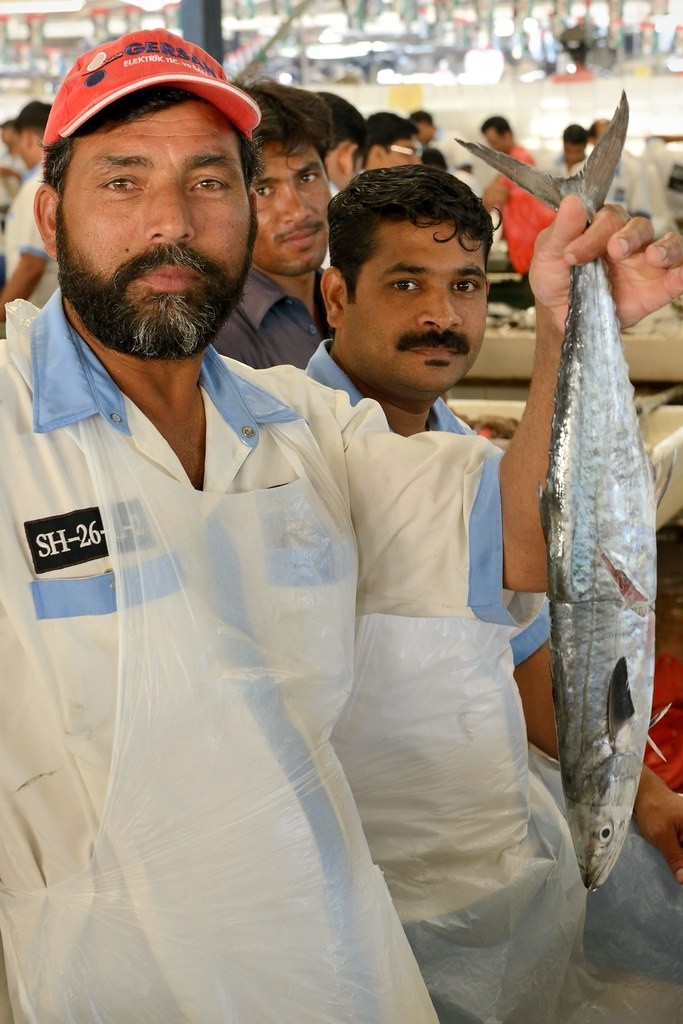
[42, 29, 262, 175]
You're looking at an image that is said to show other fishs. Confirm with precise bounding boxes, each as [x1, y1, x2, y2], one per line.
[454, 89, 656, 891]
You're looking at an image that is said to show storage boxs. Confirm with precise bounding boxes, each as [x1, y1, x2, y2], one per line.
[446, 393, 683, 532]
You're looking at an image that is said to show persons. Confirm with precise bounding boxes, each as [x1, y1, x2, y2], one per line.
[291, 159, 682, 883]
[364, 112, 422, 168]
[0, 30, 682, 1024]
[0, 101, 60, 321]
[208, 82, 336, 369]
[314, 91, 369, 268]
[555, 121, 652, 216]
[409, 111, 480, 195]
[480, 116, 556, 273]
